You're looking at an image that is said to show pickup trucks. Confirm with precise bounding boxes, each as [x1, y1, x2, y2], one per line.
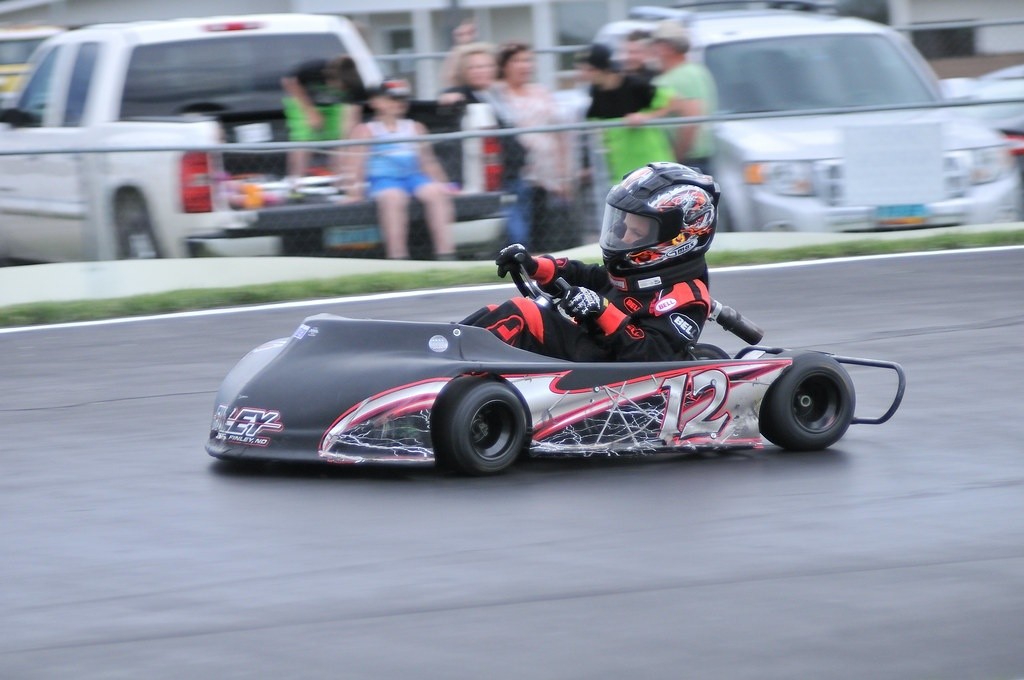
[0, 9, 518, 264]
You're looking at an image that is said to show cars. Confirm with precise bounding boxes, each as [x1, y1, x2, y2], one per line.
[539, 0, 1024, 238]
[202, 254, 911, 481]
[0, 20, 79, 123]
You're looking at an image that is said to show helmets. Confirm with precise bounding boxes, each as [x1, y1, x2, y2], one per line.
[599, 161, 721, 291]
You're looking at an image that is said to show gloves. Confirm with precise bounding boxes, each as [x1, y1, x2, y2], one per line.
[559, 287, 609, 323]
[496, 244, 539, 278]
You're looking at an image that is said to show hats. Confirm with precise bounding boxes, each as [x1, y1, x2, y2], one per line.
[380, 77, 414, 98]
[653, 20, 690, 47]
[572, 44, 611, 68]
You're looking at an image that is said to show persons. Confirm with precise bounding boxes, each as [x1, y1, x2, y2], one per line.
[277, 26, 721, 261]
[450, 161, 721, 366]
